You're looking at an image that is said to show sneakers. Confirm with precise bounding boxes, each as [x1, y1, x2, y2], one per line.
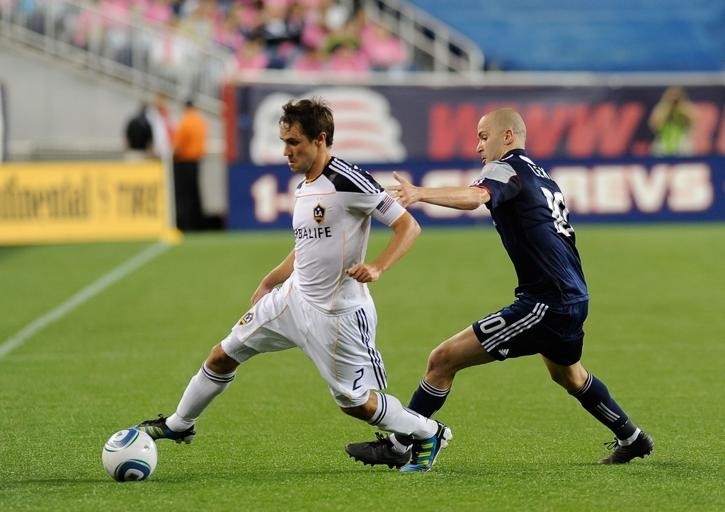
[344, 432, 412, 470]
[597, 430, 655, 464]
[398, 419, 453, 473]
[135, 414, 196, 444]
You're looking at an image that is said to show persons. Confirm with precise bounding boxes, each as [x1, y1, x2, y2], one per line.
[170, 98, 202, 231]
[149, 91, 178, 233]
[650, 82, 697, 158]
[346, 107, 655, 468]
[137, 98, 453, 472]
[0, 0, 425, 98]
[125, 102, 154, 161]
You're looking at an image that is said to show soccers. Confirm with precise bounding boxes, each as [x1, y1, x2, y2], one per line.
[102, 428, 158, 481]
